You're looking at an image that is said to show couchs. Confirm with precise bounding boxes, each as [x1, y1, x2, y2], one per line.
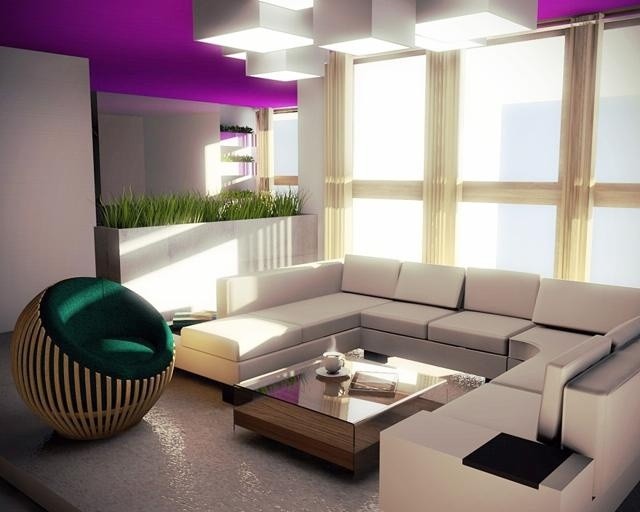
[175, 254, 640, 512]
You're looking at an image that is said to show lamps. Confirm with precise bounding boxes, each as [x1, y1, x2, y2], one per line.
[192, 0, 538, 81]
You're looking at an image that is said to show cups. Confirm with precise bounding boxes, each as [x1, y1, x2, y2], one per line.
[322, 352, 346, 374]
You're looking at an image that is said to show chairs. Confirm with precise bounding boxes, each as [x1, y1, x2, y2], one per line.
[9, 276, 177, 443]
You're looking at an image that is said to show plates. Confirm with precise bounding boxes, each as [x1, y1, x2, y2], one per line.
[315, 366, 348, 378]
